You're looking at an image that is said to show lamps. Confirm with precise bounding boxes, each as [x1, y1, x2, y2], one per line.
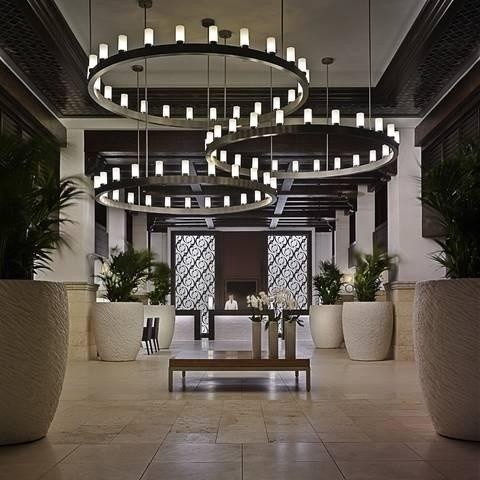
[82, 2, 400, 218]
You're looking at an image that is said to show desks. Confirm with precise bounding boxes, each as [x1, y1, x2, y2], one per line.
[169, 350, 311, 393]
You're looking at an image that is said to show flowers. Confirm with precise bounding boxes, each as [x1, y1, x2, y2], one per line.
[243, 287, 306, 329]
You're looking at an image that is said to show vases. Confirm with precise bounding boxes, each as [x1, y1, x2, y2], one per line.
[250, 321, 297, 359]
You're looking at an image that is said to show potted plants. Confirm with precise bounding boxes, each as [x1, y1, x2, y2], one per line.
[0, 120, 89, 447]
[90, 239, 176, 362]
[413, 133, 480, 443]
[308, 239, 401, 361]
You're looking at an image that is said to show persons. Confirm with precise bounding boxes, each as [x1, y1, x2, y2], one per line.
[224, 293, 238, 310]
